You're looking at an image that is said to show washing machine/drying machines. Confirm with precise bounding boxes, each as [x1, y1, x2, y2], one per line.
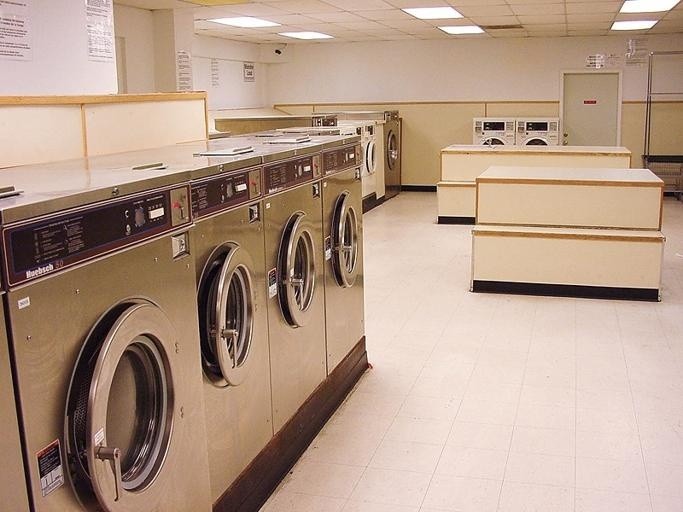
[281, 111, 401, 213]
[515, 117, 560, 145]
[472, 116, 516, 146]
[0, 132, 368, 512]
